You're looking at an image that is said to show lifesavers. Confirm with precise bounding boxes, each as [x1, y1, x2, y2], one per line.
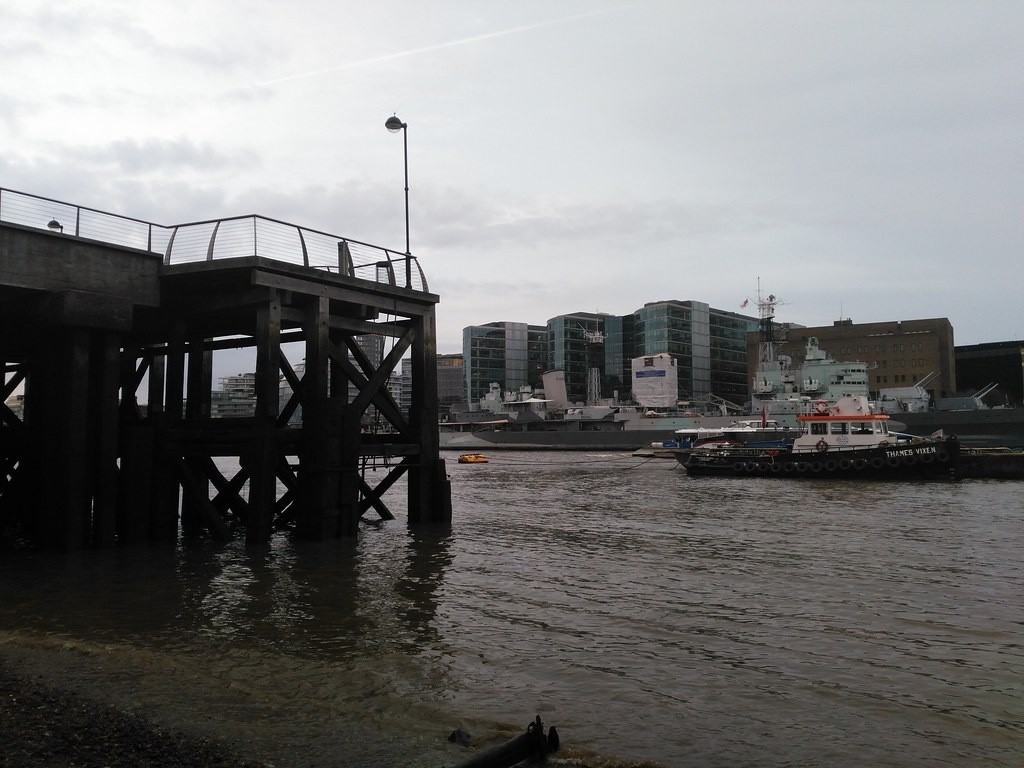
[733, 462, 744, 472]
[770, 462, 780, 472]
[870, 458, 884, 469]
[839, 459, 851, 470]
[903, 454, 916, 466]
[936, 450, 950, 461]
[757, 462, 768, 471]
[888, 456, 900, 467]
[796, 462, 808, 472]
[826, 459, 837, 471]
[745, 461, 755, 471]
[811, 462, 822, 472]
[816, 440, 829, 453]
[783, 462, 794, 472]
[815, 399, 830, 413]
[919, 452, 933, 463]
[853, 459, 865, 470]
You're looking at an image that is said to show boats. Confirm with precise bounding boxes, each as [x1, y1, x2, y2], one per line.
[458, 453, 489, 463]
[649, 397, 961, 478]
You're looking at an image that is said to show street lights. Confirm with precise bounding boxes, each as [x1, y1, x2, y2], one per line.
[386, 116, 411, 289]
